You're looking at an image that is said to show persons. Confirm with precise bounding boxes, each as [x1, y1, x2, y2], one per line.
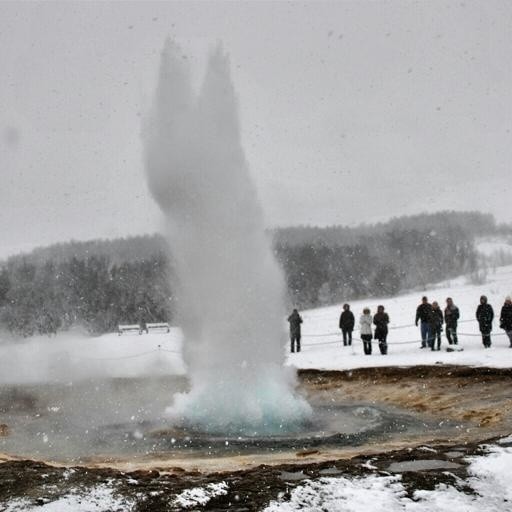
[498, 294, 512, 348]
[475, 295, 493, 347]
[287, 307, 303, 353]
[444, 297, 459, 345]
[373, 304, 390, 354]
[415, 296, 432, 348]
[339, 303, 355, 346]
[135, 305, 149, 335]
[428, 301, 443, 351]
[359, 306, 373, 355]
